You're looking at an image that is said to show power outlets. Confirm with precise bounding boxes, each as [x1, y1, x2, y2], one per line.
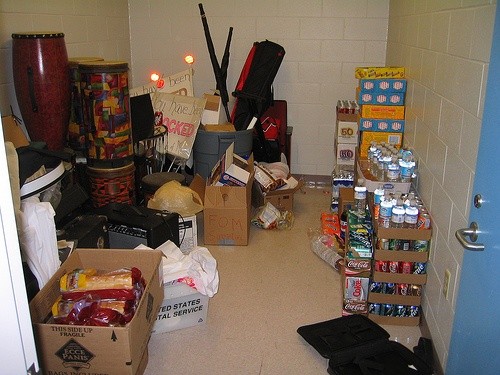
[443, 268, 451, 298]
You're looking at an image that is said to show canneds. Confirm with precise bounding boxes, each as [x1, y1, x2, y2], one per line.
[414, 196, 431, 229]
[369, 238, 429, 317]
[337, 100, 360, 114]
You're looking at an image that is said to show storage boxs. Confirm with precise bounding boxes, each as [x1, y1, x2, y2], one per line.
[321, 67, 432, 328]
[29, 142, 306, 375]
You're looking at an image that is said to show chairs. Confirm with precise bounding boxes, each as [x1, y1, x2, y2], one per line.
[254, 100, 292, 174]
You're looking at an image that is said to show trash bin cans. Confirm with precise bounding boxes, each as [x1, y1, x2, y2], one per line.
[193, 129, 255, 178]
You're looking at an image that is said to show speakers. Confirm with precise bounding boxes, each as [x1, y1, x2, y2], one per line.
[59, 202, 180, 269]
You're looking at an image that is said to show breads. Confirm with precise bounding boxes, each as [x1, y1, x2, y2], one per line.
[66, 272, 134, 314]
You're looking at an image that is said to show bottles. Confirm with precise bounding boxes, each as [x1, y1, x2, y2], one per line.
[331, 168, 353, 180]
[374, 185, 418, 229]
[339, 205, 352, 242]
[367, 141, 415, 183]
[338, 99, 360, 114]
